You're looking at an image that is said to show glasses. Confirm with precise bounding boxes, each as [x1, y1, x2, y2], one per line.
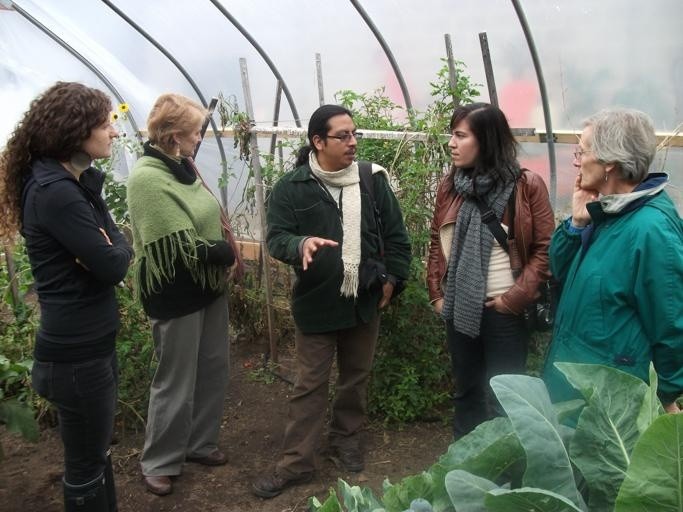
[325, 133, 363, 141]
[573, 150, 591, 160]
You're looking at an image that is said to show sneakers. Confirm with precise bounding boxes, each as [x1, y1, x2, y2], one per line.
[144, 477, 172, 495]
[334, 449, 365, 471]
[252, 471, 312, 498]
[190, 450, 227, 465]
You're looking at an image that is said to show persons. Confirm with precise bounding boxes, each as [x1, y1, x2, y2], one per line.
[0, 82, 135, 512]
[548, 107, 681, 431]
[253, 104, 412, 501]
[126, 95, 238, 496]
[428, 104, 557, 443]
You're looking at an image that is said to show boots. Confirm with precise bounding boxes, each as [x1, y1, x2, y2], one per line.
[62, 447, 118, 512]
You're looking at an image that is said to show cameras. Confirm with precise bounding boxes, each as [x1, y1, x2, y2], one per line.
[525, 302, 554, 333]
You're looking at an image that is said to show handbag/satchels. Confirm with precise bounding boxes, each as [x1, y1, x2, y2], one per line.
[358, 260, 406, 302]
[523, 279, 555, 332]
